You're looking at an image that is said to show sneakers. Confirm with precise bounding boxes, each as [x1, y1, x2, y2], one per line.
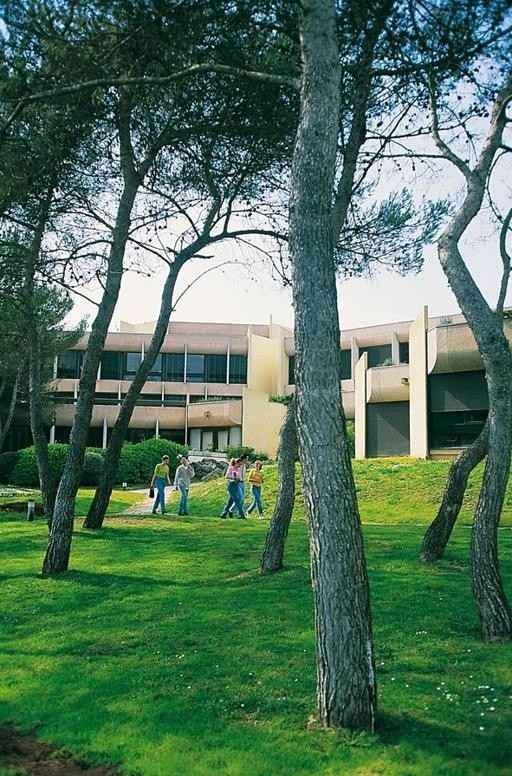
[221, 510, 265, 519]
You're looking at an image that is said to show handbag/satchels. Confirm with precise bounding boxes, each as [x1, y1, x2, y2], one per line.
[149, 486, 154, 497]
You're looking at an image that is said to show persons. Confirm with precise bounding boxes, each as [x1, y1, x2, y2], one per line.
[150, 455, 172, 517]
[220, 457, 247, 519]
[244, 460, 266, 521]
[174, 455, 196, 516]
[228, 454, 248, 518]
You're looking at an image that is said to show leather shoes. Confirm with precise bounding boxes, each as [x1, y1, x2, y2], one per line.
[152, 510, 188, 515]
